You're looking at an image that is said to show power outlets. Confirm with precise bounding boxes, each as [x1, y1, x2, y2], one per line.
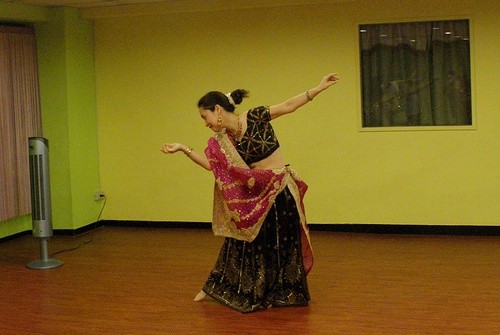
[93, 192, 107, 201]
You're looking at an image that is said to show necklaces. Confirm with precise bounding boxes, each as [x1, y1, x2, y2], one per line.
[226, 114, 243, 140]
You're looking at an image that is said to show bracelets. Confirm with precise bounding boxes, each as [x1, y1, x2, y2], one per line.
[183, 146, 193, 157]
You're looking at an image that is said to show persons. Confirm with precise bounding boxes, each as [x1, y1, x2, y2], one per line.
[159, 71, 342, 314]
